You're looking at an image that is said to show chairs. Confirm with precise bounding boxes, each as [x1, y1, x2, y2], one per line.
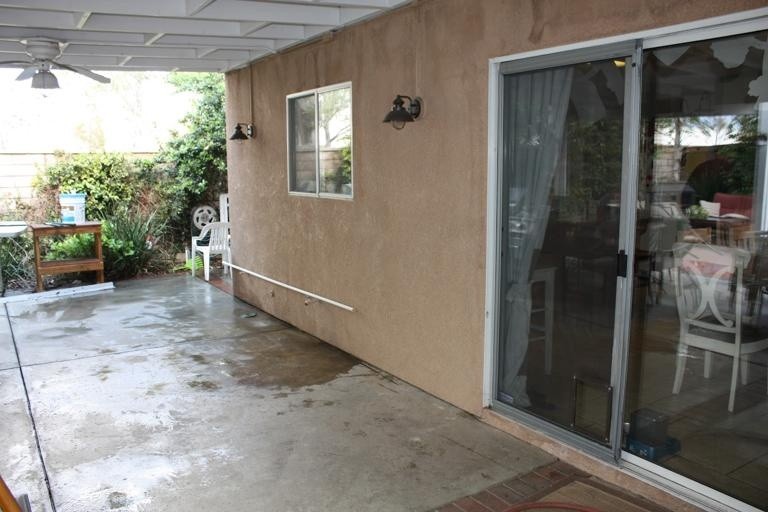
[191, 220, 232, 282]
[671, 240, 768, 413]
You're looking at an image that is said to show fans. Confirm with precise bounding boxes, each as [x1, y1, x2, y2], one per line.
[0, 37, 112, 84]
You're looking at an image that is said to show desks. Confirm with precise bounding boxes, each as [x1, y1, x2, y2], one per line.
[528, 259, 560, 375]
[29, 220, 106, 293]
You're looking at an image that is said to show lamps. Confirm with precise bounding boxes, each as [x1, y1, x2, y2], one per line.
[381, 94, 425, 130]
[229, 122, 255, 143]
[30, 64, 60, 89]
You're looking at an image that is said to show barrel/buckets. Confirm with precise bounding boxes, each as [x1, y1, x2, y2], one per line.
[59, 193, 86, 224]
